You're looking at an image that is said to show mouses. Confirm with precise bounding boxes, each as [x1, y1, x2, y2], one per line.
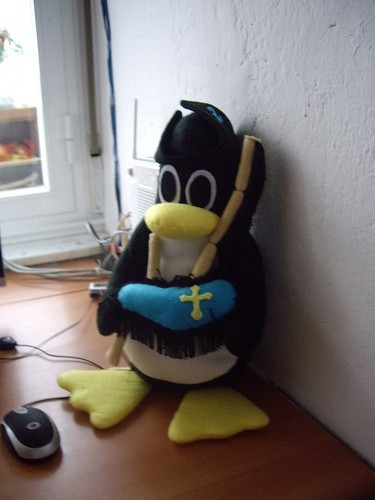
[0, 406, 64, 460]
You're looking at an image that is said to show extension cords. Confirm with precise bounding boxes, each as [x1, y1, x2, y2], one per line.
[89, 282, 109, 298]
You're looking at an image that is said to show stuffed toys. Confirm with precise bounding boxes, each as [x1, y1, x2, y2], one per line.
[57, 100, 271, 445]
